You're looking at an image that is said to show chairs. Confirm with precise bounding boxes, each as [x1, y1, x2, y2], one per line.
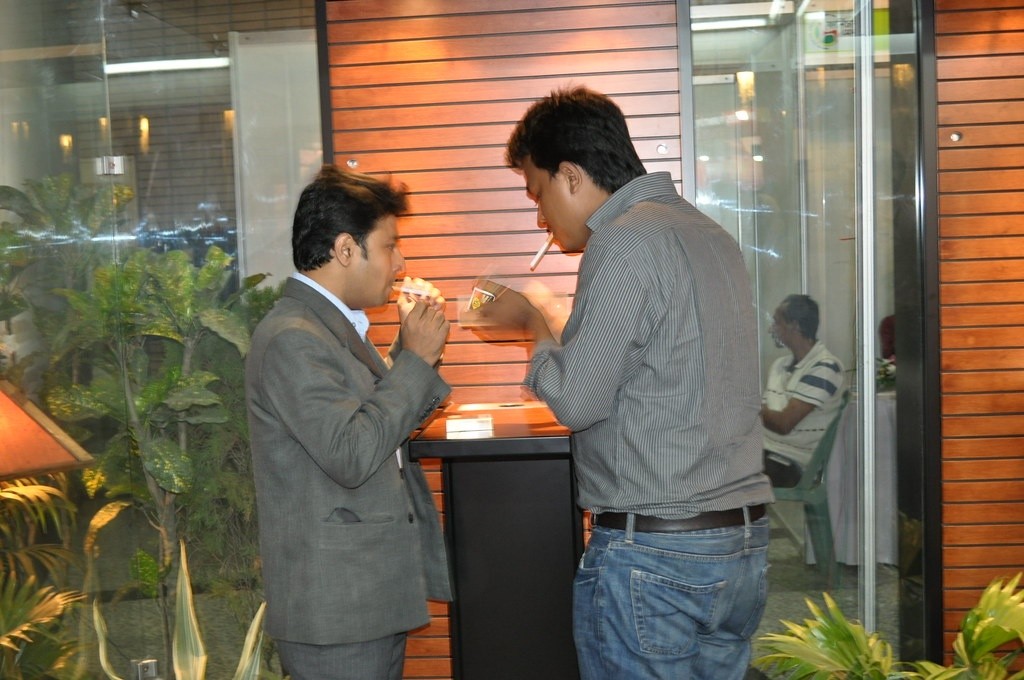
[770, 387, 853, 592]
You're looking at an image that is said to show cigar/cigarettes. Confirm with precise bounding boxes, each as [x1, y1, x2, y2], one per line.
[530, 231, 554, 272]
[392, 285, 426, 295]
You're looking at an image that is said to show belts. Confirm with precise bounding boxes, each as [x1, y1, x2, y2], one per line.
[590, 504, 766, 532]
[765, 452, 802, 478]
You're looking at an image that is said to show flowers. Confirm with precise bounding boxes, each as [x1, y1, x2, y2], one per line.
[874, 354, 897, 392]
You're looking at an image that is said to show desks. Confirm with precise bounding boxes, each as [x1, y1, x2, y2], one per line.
[804, 387, 904, 567]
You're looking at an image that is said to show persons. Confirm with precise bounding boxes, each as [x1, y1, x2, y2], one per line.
[760, 293, 848, 488]
[469, 84, 779, 680]
[243, 167, 456, 680]
